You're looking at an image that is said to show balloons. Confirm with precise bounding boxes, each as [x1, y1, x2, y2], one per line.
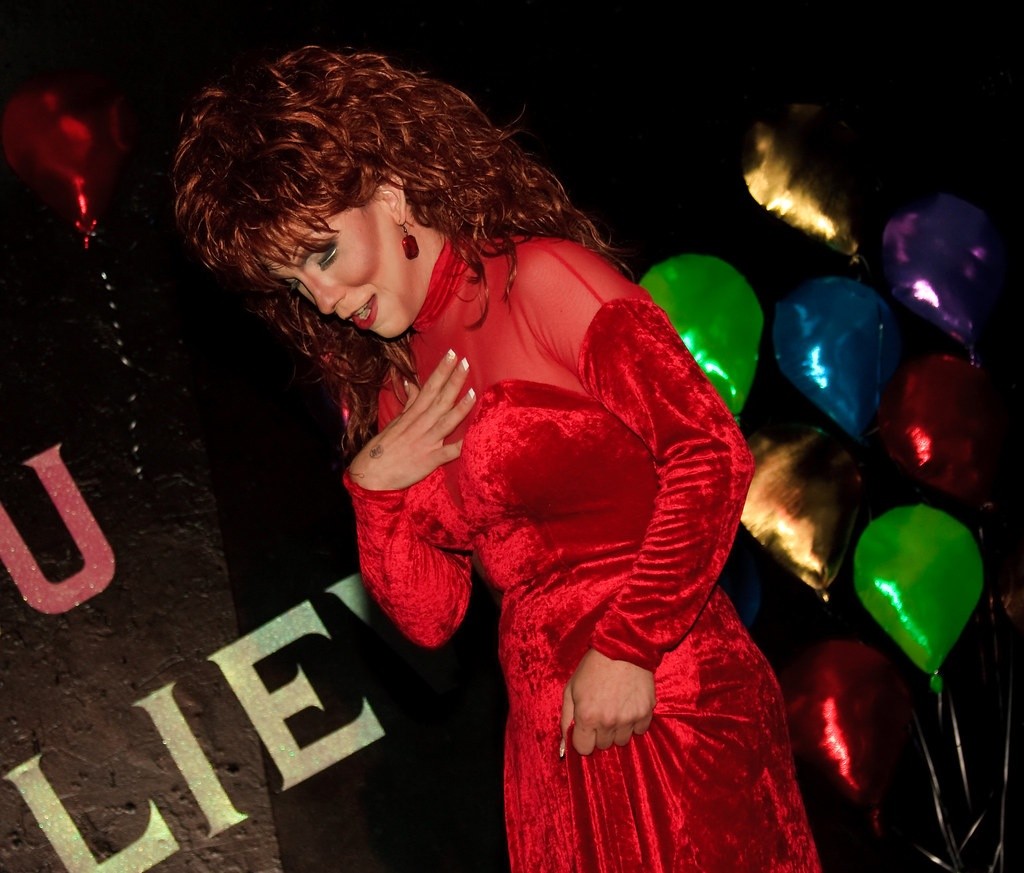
[2, 71, 137, 250]
[641, 101, 1023, 838]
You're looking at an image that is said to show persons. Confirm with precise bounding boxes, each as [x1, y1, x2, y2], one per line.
[171, 45, 823, 873]
